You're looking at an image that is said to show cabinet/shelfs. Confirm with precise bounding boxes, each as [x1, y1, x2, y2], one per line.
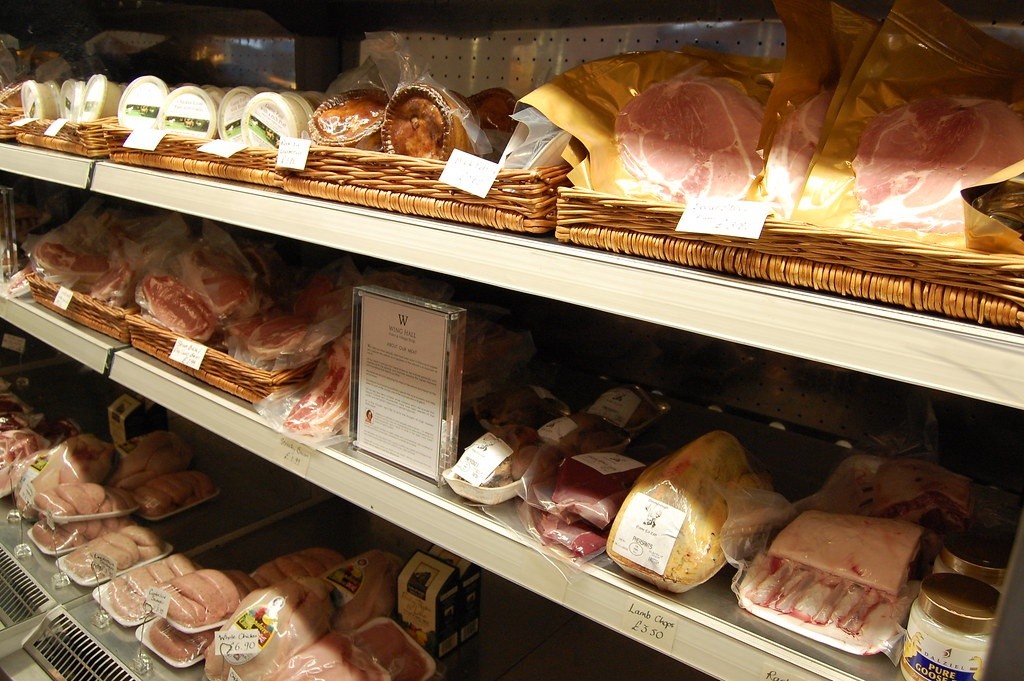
[0, 143, 1024, 681]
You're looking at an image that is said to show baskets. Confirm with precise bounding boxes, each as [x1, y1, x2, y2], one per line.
[101, 123, 284, 186]
[0, 108, 25, 138]
[12, 114, 133, 158]
[282, 143, 572, 234]
[23, 270, 142, 343]
[555, 185, 1024, 333]
[126, 314, 330, 408]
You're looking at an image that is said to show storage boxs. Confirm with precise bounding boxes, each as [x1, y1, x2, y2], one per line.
[106, 390, 169, 446]
[394, 554, 460, 658]
[428, 545, 479, 645]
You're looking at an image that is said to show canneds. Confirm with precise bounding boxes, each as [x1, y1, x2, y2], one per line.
[900, 530, 1009, 681]
